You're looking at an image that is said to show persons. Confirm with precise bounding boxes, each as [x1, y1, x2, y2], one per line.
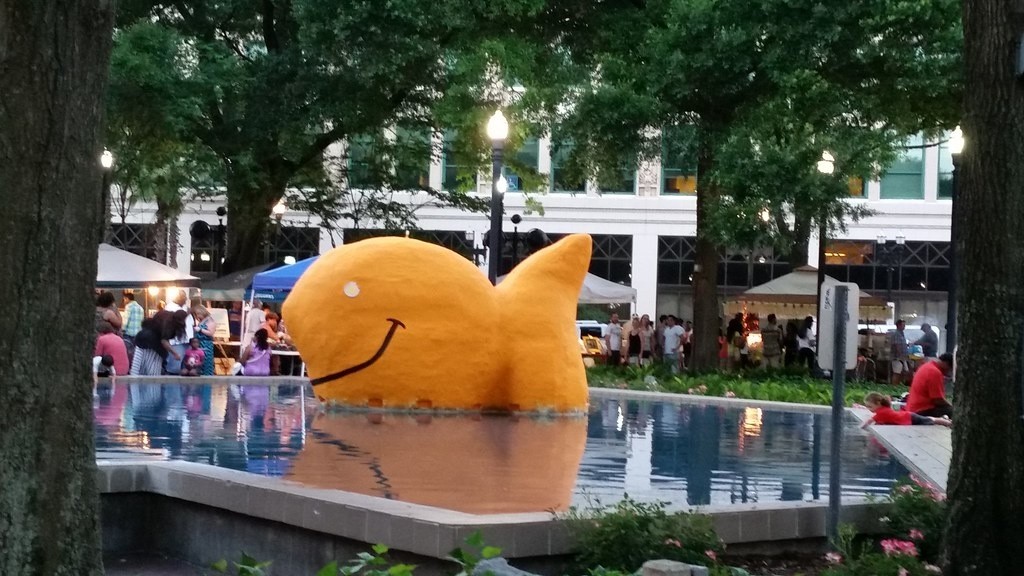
[604, 312, 954, 430]
[92, 377, 310, 446]
[94, 289, 294, 377]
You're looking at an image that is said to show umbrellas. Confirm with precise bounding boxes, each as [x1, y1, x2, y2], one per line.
[744, 266, 887, 306]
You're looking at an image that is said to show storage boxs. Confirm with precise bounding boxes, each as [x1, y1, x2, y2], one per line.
[214, 357, 235, 376]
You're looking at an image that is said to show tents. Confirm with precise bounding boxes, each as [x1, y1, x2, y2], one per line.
[95, 243, 320, 322]
[495, 270, 637, 315]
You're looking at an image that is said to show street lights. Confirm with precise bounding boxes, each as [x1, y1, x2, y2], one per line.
[876, 228, 905, 325]
[812, 150, 835, 378]
[485, 109, 508, 286]
[96, 149, 112, 244]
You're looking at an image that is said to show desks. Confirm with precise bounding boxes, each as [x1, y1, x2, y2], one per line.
[877, 354, 921, 383]
[214, 341, 242, 346]
[272, 350, 305, 377]
